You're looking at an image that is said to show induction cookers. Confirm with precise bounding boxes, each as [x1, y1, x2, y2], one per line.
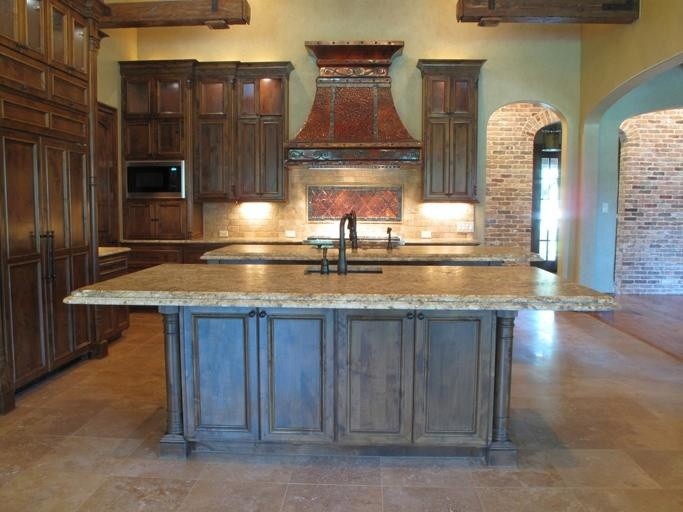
[307, 235, 400, 249]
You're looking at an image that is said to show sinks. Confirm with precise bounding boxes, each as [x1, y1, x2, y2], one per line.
[304, 265, 383, 275]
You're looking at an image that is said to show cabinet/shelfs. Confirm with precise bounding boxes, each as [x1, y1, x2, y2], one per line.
[3, 38, 46, 129]
[124, 61, 188, 155]
[198, 67, 231, 195]
[241, 69, 285, 197]
[47, 3, 95, 64]
[129, 246, 181, 267]
[337, 308, 496, 468]
[48, 67, 94, 134]
[3, 1, 47, 63]
[4, 128, 93, 398]
[123, 198, 193, 237]
[185, 246, 218, 266]
[416, 59, 487, 204]
[178, 306, 335, 459]
[96, 253, 128, 344]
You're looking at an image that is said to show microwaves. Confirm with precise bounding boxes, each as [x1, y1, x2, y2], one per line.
[125, 159, 186, 199]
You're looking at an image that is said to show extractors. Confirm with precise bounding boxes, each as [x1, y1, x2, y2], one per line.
[284, 40, 425, 170]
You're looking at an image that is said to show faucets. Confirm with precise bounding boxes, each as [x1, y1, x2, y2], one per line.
[346, 209, 359, 249]
[338, 213, 355, 274]
